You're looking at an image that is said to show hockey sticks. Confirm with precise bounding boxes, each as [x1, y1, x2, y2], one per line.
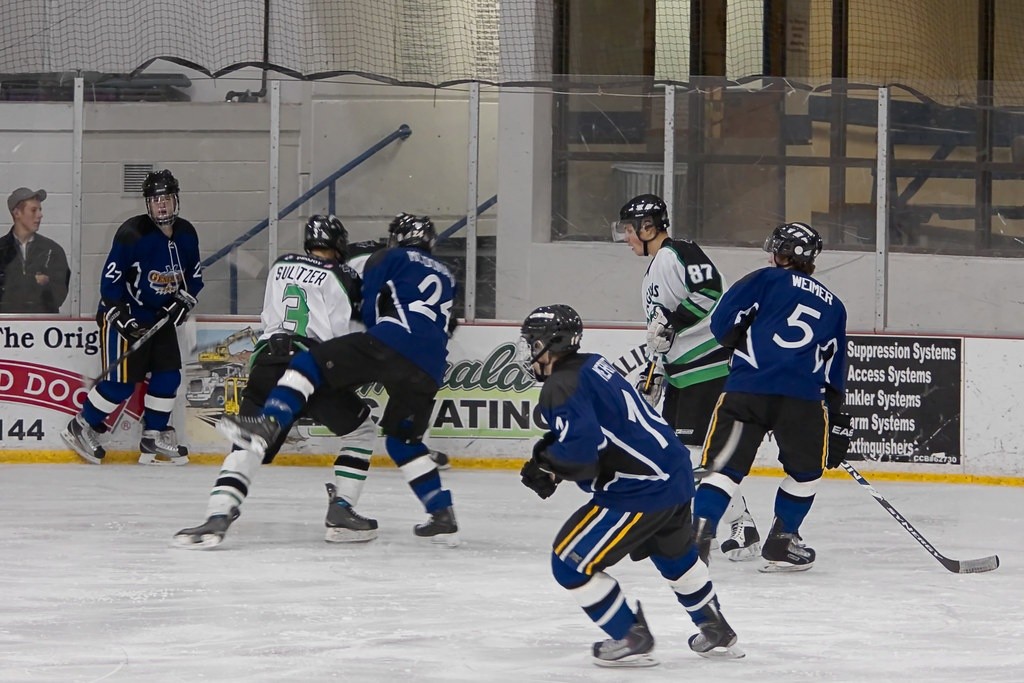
[81, 315, 170, 391]
[840, 459, 1000, 573]
[644, 351, 660, 394]
[693, 450, 714, 479]
[428, 448, 448, 466]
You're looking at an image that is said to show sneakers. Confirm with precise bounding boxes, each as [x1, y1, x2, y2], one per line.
[140, 429, 188, 466]
[593, 599, 655, 667]
[61, 414, 106, 466]
[414, 505, 458, 549]
[323, 481, 378, 544]
[686, 593, 747, 662]
[176, 514, 239, 551]
[428, 449, 449, 465]
[721, 497, 761, 561]
[759, 514, 816, 574]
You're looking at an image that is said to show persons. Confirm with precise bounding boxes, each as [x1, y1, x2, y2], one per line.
[690, 222, 854, 565]
[173, 214, 378, 540]
[68, 169, 204, 459]
[618, 193, 760, 553]
[223, 212, 459, 537]
[520, 304, 736, 662]
[0, 187, 71, 313]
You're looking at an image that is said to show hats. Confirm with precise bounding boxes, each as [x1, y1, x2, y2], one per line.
[7, 187, 47, 212]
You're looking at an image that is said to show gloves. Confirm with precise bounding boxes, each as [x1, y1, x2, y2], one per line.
[116, 317, 150, 342]
[646, 314, 674, 355]
[159, 300, 189, 327]
[828, 412, 853, 471]
[524, 466, 561, 500]
[634, 372, 665, 406]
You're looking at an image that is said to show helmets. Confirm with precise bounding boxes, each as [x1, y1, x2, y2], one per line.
[304, 215, 348, 255]
[612, 194, 671, 241]
[514, 305, 584, 383]
[387, 212, 438, 247]
[763, 222, 823, 263]
[141, 169, 182, 224]
